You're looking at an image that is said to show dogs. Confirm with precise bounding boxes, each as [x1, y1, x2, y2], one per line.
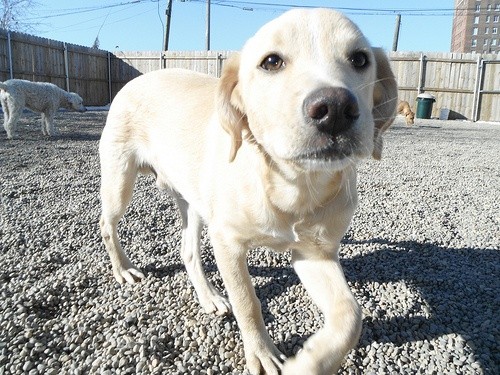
[0, 78, 87, 140]
[397, 100, 415, 126]
[98, 6, 399, 375]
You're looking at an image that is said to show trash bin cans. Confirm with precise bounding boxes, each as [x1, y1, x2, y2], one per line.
[439, 108, 450, 120]
[415, 91, 436, 119]
